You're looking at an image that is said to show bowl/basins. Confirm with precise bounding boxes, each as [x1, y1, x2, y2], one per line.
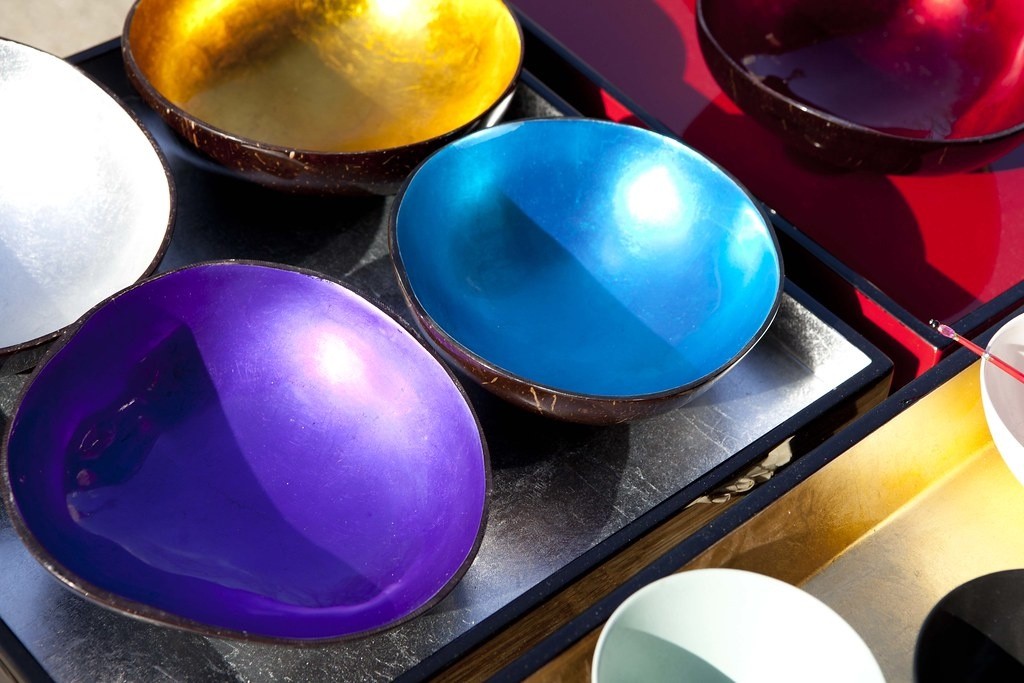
[0, 260, 493, 646]
[392, 117, 781, 420]
[122, 0, 525, 193]
[913, 569, 1024, 683]
[592, 568, 885, 683]
[979, 313, 1024, 485]
[0, 36, 177, 375]
[695, 0, 1024, 177]
